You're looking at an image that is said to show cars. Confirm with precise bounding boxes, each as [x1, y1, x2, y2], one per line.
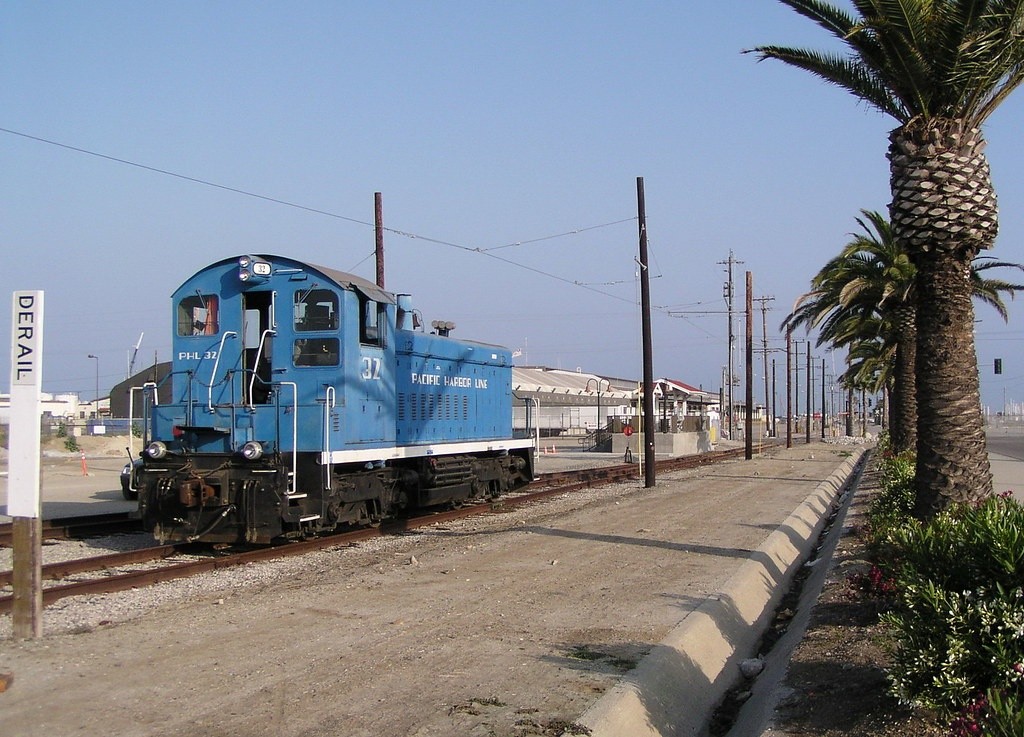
[120, 458, 143, 501]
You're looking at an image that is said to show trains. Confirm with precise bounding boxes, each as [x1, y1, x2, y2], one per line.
[138, 253, 537, 551]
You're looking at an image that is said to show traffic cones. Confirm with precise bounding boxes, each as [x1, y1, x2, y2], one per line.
[552, 445, 556, 453]
[544, 446, 548, 455]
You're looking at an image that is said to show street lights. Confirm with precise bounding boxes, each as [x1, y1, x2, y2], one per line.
[585, 377, 612, 429]
[88, 355, 98, 419]
